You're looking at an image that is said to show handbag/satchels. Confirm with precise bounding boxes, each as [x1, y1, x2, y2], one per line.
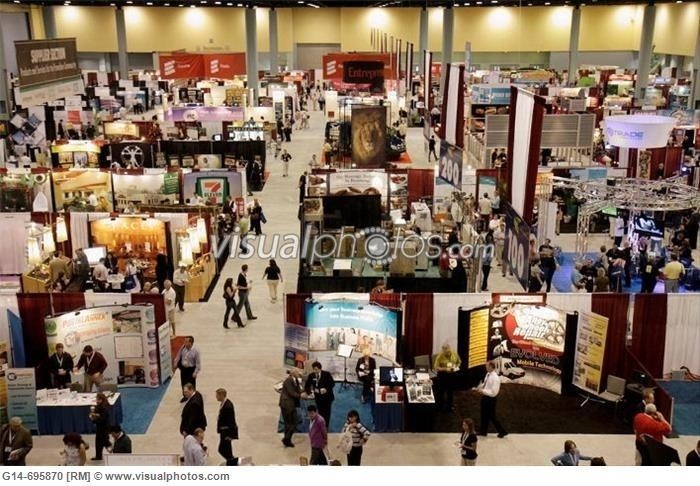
[177, 357, 183, 371]
[223, 292, 236, 301]
[337, 432, 353, 456]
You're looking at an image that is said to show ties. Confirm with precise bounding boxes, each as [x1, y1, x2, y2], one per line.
[314, 373, 320, 392]
[482, 372, 490, 390]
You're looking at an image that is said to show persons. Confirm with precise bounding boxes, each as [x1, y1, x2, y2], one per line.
[1, 77, 700, 466]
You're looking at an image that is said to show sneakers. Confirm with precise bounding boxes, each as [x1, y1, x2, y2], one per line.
[247, 316, 258, 320]
[180, 395, 188, 403]
[237, 323, 247, 328]
[231, 316, 237, 323]
[476, 429, 509, 439]
[223, 323, 230, 329]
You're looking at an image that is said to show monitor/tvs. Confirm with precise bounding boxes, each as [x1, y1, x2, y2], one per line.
[379, 366, 405, 389]
[213, 134, 221, 141]
